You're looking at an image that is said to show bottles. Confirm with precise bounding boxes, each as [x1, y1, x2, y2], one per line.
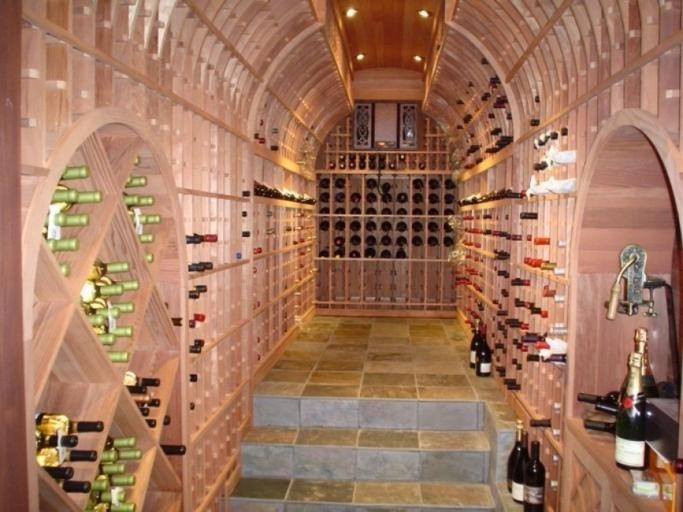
[36, 155, 217, 512]
[579, 327, 683, 475]
[455, 56, 568, 512]
[241, 118, 454, 259]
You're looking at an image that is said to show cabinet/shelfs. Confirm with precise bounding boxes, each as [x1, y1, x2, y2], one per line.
[314, 99, 461, 318]
[421, 0, 680, 510]
[0, 0, 352, 510]
